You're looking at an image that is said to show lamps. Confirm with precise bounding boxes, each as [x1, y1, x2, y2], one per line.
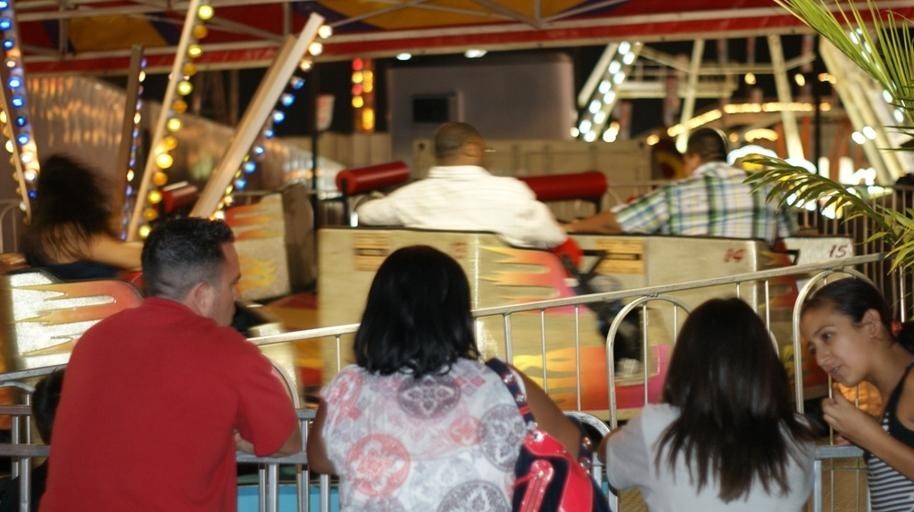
[253, 144, 263, 154]
[171, 100, 187, 114]
[193, 25, 208, 40]
[0, 19, 12, 31]
[129, 152, 137, 160]
[182, 62, 197, 76]
[126, 185, 133, 196]
[198, 5, 214, 20]
[234, 178, 246, 188]
[308, 42, 322, 55]
[12, 173, 19, 183]
[225, 186, 234, 193]
[5, 58, 16, 68]
[3, 40, 15, 49]
[270, 109, 284, 122]
[130, 145, 137, 152]
[289, 76, 304, 89]
[155, 152, 173, 169]
[13, 116, 27, 128]
[23, 169, 37, 181]
[143, 207, 158, 220]
[318, 25, 331, 39]
[261, 127, 274, 138]
[19, 151, 34, 163]
[218, 202, 225, 210]
[8, 78, 20, 88]
[9, 96, 24, 107]
[300, 55, 312, 70]
[138, 85, 144, 96]
[152, 171, 167, 185]
[134, 113, 142, 124]
[224, 196, 235, 206]
[243, 161, 256, 173]
[139, 225, 152, 237]
[140, 71, 146, 82]
[132, 128, 139, 139]
[178, 81, 192, 95]
[27, 191, 38, 199]
[129, 160, 137, 166]
[584, 131, 596, 142]
[16, 131, 29, 145]
[126, 169, 135, 183]
[363, 71, 373, 80]
[166, 117, 181, 131]
[280, 93, 294, 106]
[187, 44, 202, 58]
[137, 99, 143, 110]
[163, 134, 177, 150]
[147, 189, 161, 204]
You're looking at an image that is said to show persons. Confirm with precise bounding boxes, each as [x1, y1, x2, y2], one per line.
[562, 128, 802, 248]
[5, 370, 64, 512]
[307, 245, 579, 512]
[800, 280, 914, 512]
[355, 121, 643, 379]
[39, 217, 297, 511]
[598, 297, 812, 510]
[20, 153, 270, 336]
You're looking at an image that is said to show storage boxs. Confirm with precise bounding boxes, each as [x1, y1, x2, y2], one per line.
[20, 203, 27, 211]
[362, 110, 374, 119]
[215, 210, 226, 219]
[351, 95, 365, 109]
[244, 155, 251, 162]
[141, 59, 147, 68]
[593, 111, 606, 124]
[623, 54, 635, 65]
[352, 71, 363, 82]
[587, 100, 602, 113]
[352, 58, 363, 69]
[237, 169, 242, 177]
[16, 188, 23, 197]
[607, 61, 622, 73]
[604, 91, 616, 104]
[9, 157, 16, 167]
[0, 111, 8, 124]
[463, 48, 487, 57]
[363, 119, 375, 130]
[613, 72, 626, 83]
[571, 129, 579, 138]
[352, 85, 361, 92]
[22, 216, 29, 227]
[398, 53, 412, 61]
[364, 81, 372, 93]
[580, 122, 591, 133]
[3, 126, 10, 138]
[0, 1, 8, 10]
[5, 140, 14, 154]
[615, 40, 629, 54]
[597, 81, 611, 93]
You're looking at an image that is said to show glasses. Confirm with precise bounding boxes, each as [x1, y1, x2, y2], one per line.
[466, 139, 495, 157]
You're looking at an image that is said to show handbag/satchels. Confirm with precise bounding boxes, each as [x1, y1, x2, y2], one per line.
[485, 357, 612, 512]
